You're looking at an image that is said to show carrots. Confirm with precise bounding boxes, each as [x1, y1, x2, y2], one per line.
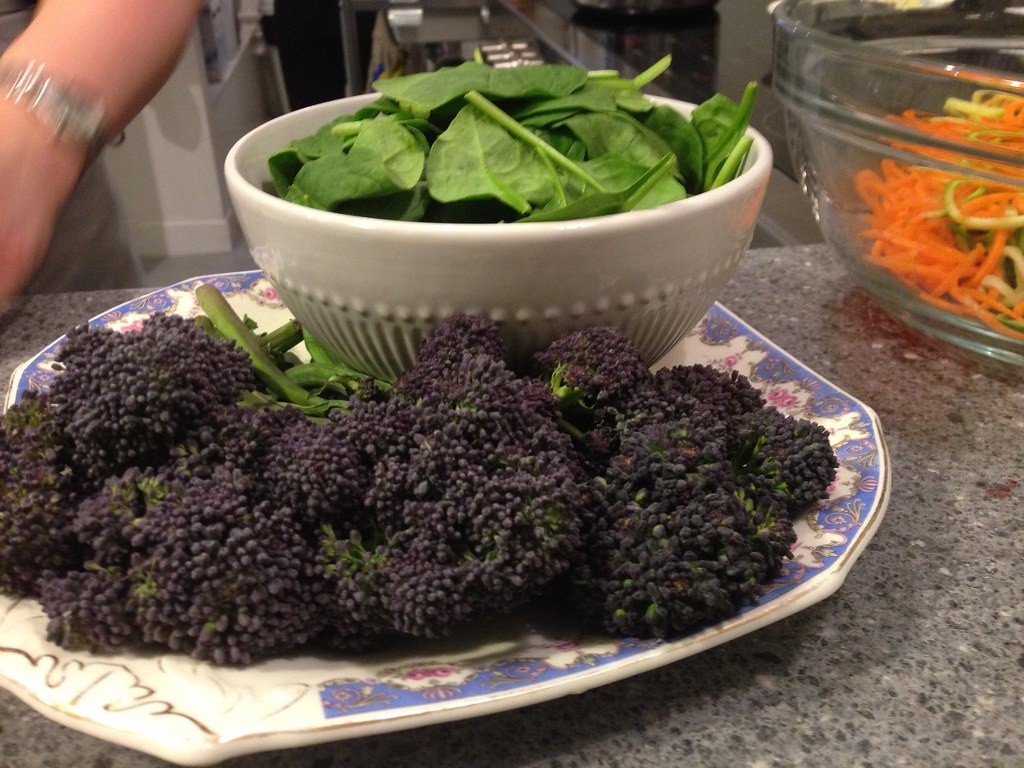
[855, 98, 1024, 338]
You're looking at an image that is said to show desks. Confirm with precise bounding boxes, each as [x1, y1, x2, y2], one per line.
[1, 243, 1024, 767]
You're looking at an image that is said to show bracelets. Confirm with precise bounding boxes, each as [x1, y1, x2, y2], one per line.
[0, 62, 108, 165]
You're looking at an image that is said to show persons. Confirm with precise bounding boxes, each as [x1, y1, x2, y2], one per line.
[0, 0, 207, 311]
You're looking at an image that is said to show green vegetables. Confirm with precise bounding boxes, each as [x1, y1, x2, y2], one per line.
[269, 59, 757, 223]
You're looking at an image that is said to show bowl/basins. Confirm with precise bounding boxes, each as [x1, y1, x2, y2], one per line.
[771, 0, 1024, 384]
[223, 92, 773, 387]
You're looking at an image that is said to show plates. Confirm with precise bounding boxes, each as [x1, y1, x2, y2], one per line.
[0, 270, 892, 765]
